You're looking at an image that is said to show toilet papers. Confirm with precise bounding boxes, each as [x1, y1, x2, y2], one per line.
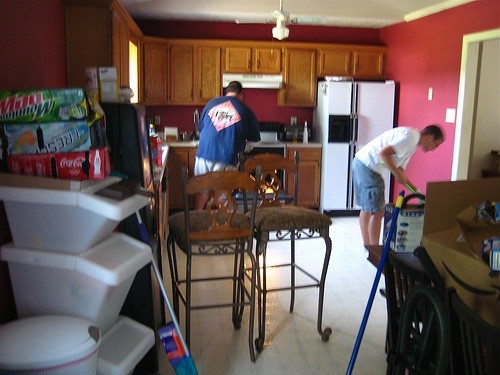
[242, 82, 279, 88]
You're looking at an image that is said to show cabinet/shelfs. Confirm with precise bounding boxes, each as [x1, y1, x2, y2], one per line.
[286, 147, 321, 211]
[277, 48, 318, 107]
[223, 41, 283, 74]
[167, 147, 196, 210]
[317, 49, 387, 80]
[63, 0, 223, 106]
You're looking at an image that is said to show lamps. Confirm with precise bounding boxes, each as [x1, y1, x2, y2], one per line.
[271, 20, 290, 54]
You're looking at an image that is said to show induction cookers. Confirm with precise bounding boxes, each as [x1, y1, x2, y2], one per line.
[247, 122, 284, 143]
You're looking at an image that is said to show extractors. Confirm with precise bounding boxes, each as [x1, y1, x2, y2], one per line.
[222, 73, 283, 88]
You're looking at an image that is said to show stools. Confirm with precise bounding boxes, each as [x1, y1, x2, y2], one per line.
[238, 151, 333, 353]
[167, 159, 263, 364]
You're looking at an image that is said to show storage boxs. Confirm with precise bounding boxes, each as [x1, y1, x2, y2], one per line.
[0, 67, 157, 375]
[420, 176, 500, 333]
[384, 203, 425, 253]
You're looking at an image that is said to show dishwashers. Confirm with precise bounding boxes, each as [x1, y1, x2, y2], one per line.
[234, 143, 286, 199]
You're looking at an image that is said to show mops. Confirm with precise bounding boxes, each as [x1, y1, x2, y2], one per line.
[346, 191, 406, 375]
[136, 210, 198, 375]
[404, 181, 425, 201]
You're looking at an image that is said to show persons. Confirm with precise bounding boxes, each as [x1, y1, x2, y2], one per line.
[194, 81, 261, 210]
[351, 125, 444, 245]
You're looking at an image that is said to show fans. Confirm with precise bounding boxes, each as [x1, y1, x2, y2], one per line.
[234, 0, 329, 25]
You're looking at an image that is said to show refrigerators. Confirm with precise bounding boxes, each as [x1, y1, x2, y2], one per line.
[312, 75, 396, 217]
